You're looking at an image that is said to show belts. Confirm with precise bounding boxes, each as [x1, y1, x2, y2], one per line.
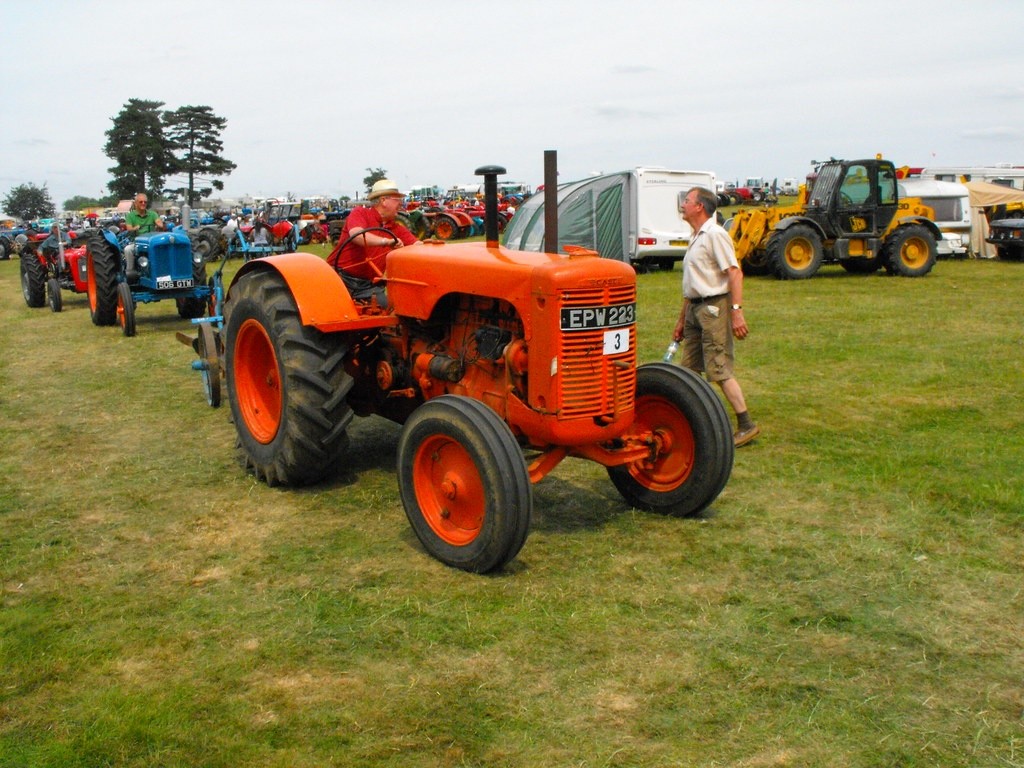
[691, 296, 714, 304]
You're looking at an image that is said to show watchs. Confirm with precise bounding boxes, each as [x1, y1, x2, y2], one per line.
[731, 304, 741, 310]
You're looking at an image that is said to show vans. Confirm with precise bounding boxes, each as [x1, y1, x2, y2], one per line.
[840, 176, 974, 264]
[566, 165, 719, 276]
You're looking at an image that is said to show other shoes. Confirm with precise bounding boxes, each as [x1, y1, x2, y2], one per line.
[733, 424, 759, 448]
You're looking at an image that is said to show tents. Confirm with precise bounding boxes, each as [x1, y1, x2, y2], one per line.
[963, 182, 1024, 259]
[501, 171, 630, 265]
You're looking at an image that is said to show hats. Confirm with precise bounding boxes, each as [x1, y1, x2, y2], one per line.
[368, 180, 409, 200]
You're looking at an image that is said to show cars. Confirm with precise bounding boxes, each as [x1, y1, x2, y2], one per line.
[985, 212, 1024, 263]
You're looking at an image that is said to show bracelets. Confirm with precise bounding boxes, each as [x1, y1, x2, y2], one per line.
[381, 238, 386, 247]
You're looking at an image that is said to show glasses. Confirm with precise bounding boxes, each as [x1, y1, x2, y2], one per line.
[139, 201, 147, 203]
[388, 197, 404, 202]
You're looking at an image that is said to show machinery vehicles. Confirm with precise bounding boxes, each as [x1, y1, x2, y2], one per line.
[724, 153, 943, 281]
[0, 178, 540, 338]
[182, 150, 735, 584]
[719, 174, 798, 216]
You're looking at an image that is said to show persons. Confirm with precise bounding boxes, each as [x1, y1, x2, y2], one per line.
[37, 222, 71, 256]
[327, 180, 420, 310]
[125, 193, 163, 238]
[671, 186, 760, 449]
